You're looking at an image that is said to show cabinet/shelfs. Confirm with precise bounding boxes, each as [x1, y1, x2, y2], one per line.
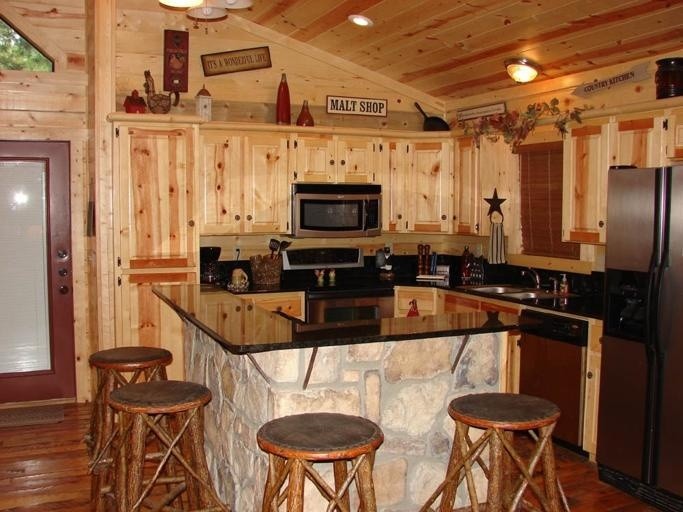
[452, 133, 518, 237]
[198, 128, 292, 235]
[251, 292, 304, 322]
[435, 288, 523, 394]
[666, 105, 682, 162]
[110, 119, 200, 423]
[290, 132, 382, 185]
[381, 136, 407, 234]
[407, 137, 453, 236]
[559, 109, 672, 247]
[392, 285, 437, 318]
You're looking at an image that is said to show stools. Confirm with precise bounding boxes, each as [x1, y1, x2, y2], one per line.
[81, 347, 229, 510]
[256, 413, 384, 511]
[417, 392, 570, 511]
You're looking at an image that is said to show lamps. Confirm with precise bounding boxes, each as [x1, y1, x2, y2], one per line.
[503, 58, 542, 83]
[159, 0, 254, 31]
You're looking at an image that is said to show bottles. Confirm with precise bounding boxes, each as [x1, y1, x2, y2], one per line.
[276, 73, 291, 125]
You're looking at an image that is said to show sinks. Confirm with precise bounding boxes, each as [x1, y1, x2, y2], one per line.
[455, 283, 530, 295]
[495, 289, 579, 302]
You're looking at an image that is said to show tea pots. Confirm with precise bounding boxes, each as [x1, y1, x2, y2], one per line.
[231, 268, 250, 288]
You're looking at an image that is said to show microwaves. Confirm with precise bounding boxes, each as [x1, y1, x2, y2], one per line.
[293, 184, 379, 233]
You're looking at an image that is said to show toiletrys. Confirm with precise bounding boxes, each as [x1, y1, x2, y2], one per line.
[558, 273, 571, 296]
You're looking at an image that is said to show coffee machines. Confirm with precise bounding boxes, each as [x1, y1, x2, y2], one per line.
[200, 246, 221, 284]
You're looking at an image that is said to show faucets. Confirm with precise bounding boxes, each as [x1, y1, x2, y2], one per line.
[547, 275, 559, 292]
[520, 266, 542, 291]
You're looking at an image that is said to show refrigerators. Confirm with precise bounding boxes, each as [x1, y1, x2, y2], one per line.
[595, 165, 683, 510]
